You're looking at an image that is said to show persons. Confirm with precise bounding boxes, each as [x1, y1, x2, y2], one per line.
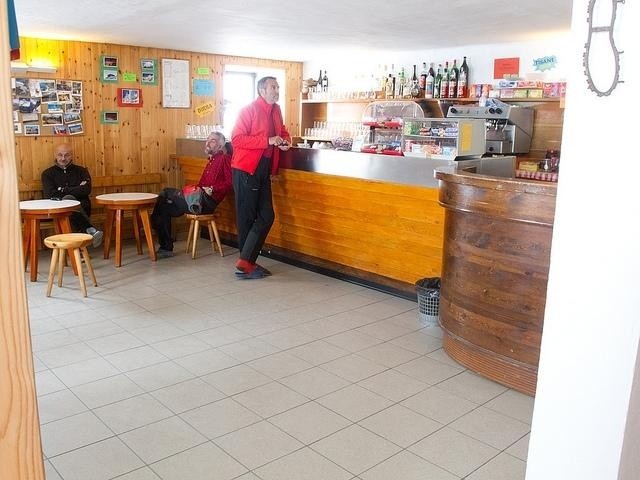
[41, 144, 103, 264]
[230, 77, 292, 279]
[140, 131, 233, 258]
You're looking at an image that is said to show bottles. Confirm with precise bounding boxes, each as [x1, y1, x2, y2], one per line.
[426, 57, 469, 99]
[317, 69, 322, 92]
[410, 64, 418, 96]
[322, 69, 328, 92]
[389, 63, 396, 95]
[381, 63, 388, 92]
[385, 73, 395, 96]
[399, 66, 407, 98]
[420, 62, 426, 89]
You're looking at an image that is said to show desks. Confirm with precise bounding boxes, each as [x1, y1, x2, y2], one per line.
[95, 192, 160, 269]
[19, 199, 82, 282]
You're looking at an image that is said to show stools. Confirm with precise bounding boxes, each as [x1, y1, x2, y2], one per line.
[44, 232, 98, 298]
[186, 211, 224, 260]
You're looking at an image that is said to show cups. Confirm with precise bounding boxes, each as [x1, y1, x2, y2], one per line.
[307, 91, 355, 99]
[313, 121, 363, 129]
[305, 127, 362, 139]
[309, 88, 313, 93]
[184, 125, 224, 139]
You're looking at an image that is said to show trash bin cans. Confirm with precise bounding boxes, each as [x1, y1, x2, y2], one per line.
[415, 277, 441, 329]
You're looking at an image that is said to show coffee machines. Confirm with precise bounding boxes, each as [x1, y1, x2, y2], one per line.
[447, 105, 535, 153]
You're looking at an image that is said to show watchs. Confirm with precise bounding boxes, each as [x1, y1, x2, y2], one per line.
[208, 187, 213, 194]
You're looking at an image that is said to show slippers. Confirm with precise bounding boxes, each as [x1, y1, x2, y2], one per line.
[93, 230, 103, 249]
[255, 266, 272, 276]
[235, 268, 264, 279]
